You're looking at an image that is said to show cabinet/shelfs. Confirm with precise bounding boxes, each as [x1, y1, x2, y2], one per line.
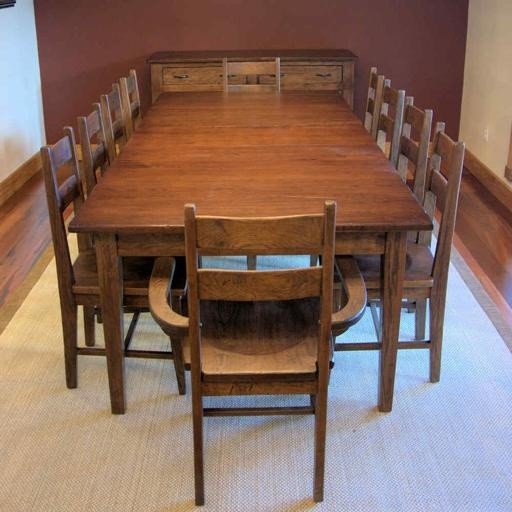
[147, 48, 358, 111]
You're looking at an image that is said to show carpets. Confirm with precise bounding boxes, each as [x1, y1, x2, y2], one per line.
[2, 217, 511, 512]
